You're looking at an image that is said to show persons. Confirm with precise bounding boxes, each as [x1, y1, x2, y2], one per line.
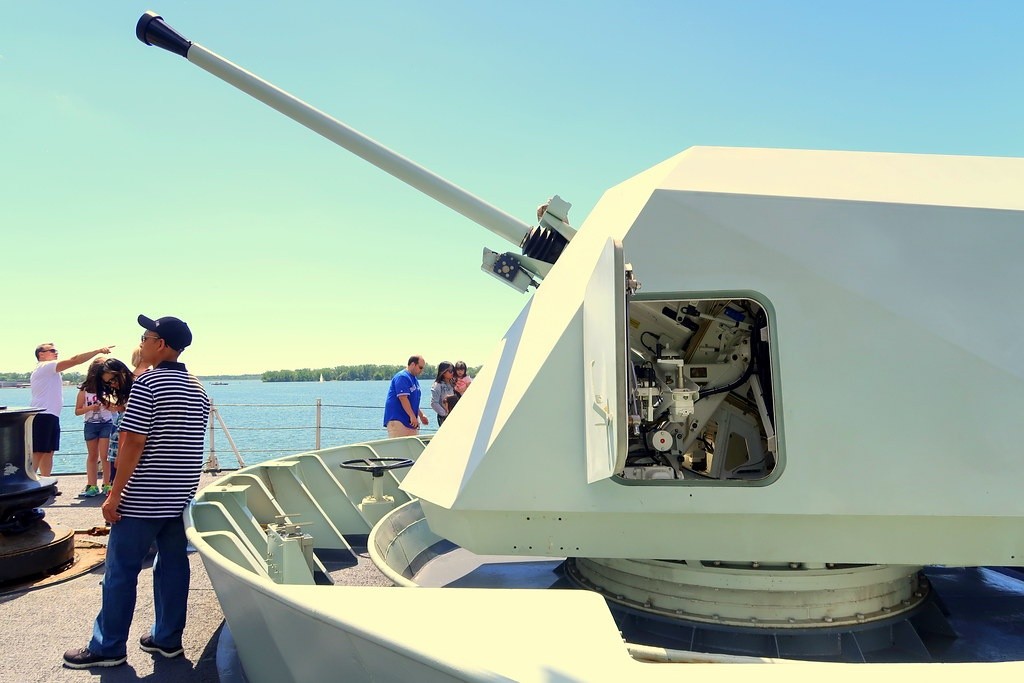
[382, 356, 430, 439]
[75, 348, 151, 498]
[29, 343, 116, 496]
[430, 361, 472, 428]
[62, 313, 211, 669]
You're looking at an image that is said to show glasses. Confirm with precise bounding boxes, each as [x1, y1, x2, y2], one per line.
[141, 335, 168, 349]
[449, 369, 454, 373]
[105, 373, 117, 387]
[414, 362, 423, 369]
[38, 349, 58, 353]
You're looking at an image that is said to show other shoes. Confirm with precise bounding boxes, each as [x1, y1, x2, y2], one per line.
[55, 492, 62, 496]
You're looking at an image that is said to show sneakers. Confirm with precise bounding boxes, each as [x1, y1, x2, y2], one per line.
[139, 632, 184, 658]
[78, 485, 101, 498]
[101, 484, 112, 496]
[62, 645, 127, 669]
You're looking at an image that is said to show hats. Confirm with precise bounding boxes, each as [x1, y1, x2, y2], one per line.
[138, 314, 192, 351]
